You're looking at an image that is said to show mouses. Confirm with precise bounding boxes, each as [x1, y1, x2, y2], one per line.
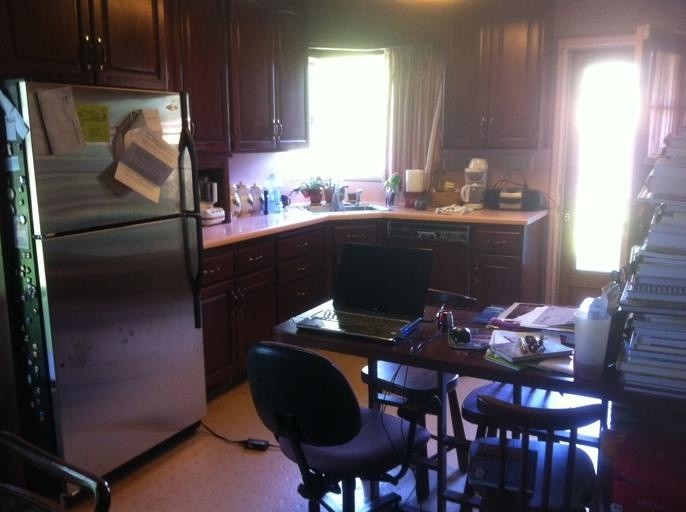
[450, 326, 471, 344]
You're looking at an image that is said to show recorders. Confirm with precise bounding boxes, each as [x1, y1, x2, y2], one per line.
[485, 189, 547, 210]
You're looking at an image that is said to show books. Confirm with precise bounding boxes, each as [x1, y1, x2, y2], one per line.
[483, 330, 574, 378]
[614, 125, 686, 402]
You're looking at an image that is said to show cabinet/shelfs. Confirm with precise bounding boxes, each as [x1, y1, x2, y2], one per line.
[168, 0, 232, 223]
[331, 221, 385, 249]
[386, 219, 471, 250]
[469, 217, 545, 304]
[277, 223, 331, 319]
[441, 0, 554, 149]
[0, 0, 168, 91]
[227, 0, 310, 153]
[202, 232, 277, 405]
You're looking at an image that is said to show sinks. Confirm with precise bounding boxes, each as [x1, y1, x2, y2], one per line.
[297, 203, 394, 213]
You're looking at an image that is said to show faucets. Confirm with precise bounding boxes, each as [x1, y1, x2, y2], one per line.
[329, 186, 349, 208]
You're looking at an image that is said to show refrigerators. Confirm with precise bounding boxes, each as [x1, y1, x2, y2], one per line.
[0, 76, 209, 500]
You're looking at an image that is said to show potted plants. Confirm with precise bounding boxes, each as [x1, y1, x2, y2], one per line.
[290, 177, 349, 203]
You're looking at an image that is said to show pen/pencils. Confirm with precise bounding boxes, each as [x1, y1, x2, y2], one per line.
[519, 337, 529, 353]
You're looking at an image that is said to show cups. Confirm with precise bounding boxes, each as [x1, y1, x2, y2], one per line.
[572, 309, 613, 382]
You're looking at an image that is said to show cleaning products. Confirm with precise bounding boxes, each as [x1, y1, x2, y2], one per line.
[266, 174, 283, 214]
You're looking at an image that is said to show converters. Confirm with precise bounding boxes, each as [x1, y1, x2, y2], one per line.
[245, 439, 268, 450]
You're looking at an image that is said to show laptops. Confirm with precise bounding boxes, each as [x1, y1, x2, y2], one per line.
[297, 242, 434, 343]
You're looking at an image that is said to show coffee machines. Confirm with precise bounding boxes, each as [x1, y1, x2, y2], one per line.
[460, 157, 489, 210]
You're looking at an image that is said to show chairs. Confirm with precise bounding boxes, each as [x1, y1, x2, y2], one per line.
[469, 396, 609, 512]
[0, 429, 111, 512]
[362, 288, 477, 512]
[462, 380, 564, 512]
[248, 342, 431, 512]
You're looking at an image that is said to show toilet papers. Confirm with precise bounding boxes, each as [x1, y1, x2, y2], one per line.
[406, 169, 424, 193]
